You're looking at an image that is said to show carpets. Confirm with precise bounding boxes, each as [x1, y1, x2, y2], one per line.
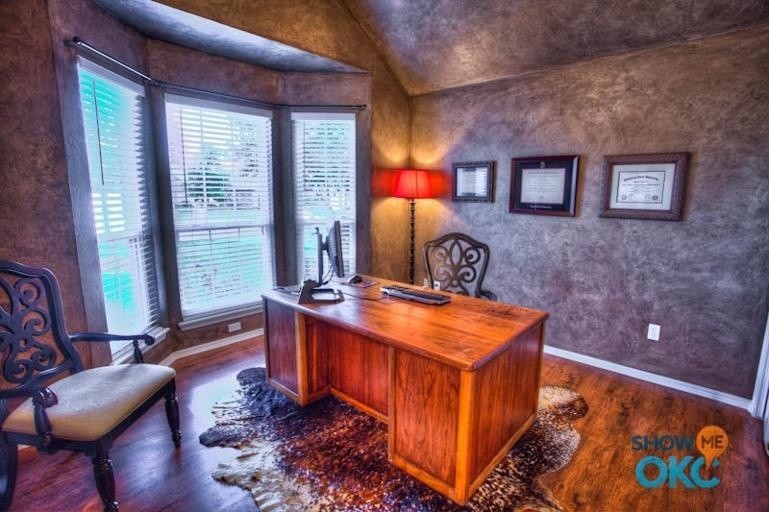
[199, 367, 588, 512]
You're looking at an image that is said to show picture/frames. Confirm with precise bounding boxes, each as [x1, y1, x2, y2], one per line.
[452, 152, 688, 220]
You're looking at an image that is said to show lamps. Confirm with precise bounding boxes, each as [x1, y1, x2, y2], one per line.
[391, 168, 432, 286]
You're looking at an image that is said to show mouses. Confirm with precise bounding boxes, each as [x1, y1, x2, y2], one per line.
[349, 274, 362, 284]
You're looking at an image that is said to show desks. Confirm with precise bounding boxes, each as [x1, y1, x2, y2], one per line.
[261, 271, 550, 507]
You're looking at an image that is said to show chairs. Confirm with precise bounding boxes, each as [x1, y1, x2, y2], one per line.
[0, 259, 181, 511]
[422, 233, 497, 301]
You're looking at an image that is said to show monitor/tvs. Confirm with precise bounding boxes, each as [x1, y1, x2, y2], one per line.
[297, 219, 345, 304]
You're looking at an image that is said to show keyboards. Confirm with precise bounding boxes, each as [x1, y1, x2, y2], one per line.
[380, 284, 452, 306]
